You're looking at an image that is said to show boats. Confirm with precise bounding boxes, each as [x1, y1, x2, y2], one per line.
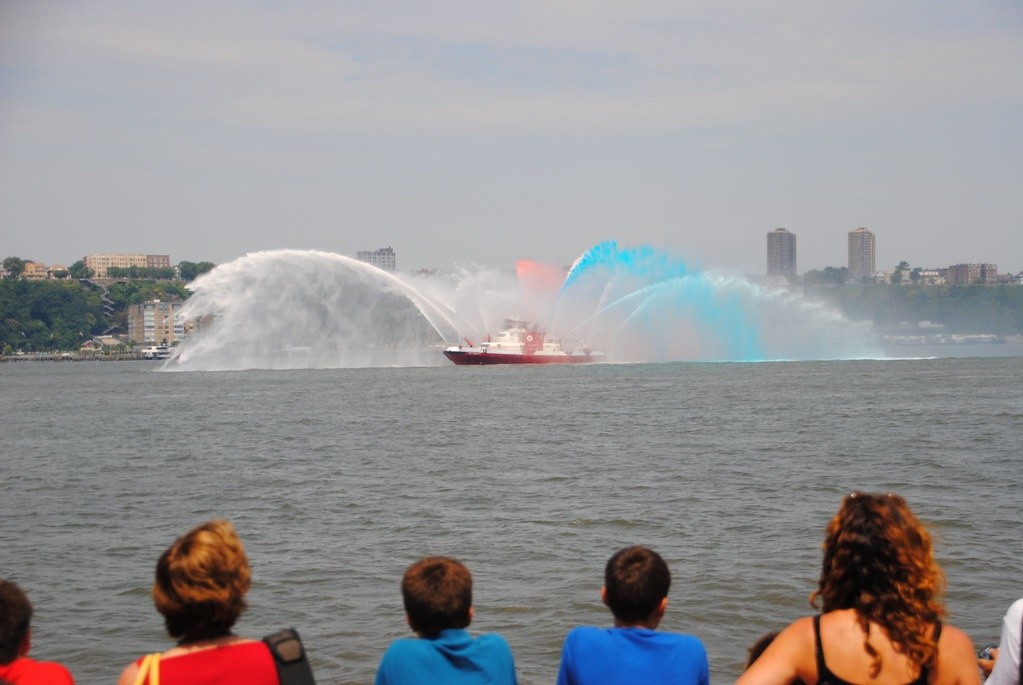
[443, 318, 604, 365]
[142, 346, 170, 360]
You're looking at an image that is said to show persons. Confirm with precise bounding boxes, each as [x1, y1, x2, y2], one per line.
[115, 518, 280, 685]
[374, 557, 518, 685]
[0, 578, 77, 685]
[735, 491, 983, 685]
[556, 546, 710, 685]
[978, 598, 1023, 685]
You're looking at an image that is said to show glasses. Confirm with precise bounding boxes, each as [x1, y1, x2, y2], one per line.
[844, 491, 903, 511]
